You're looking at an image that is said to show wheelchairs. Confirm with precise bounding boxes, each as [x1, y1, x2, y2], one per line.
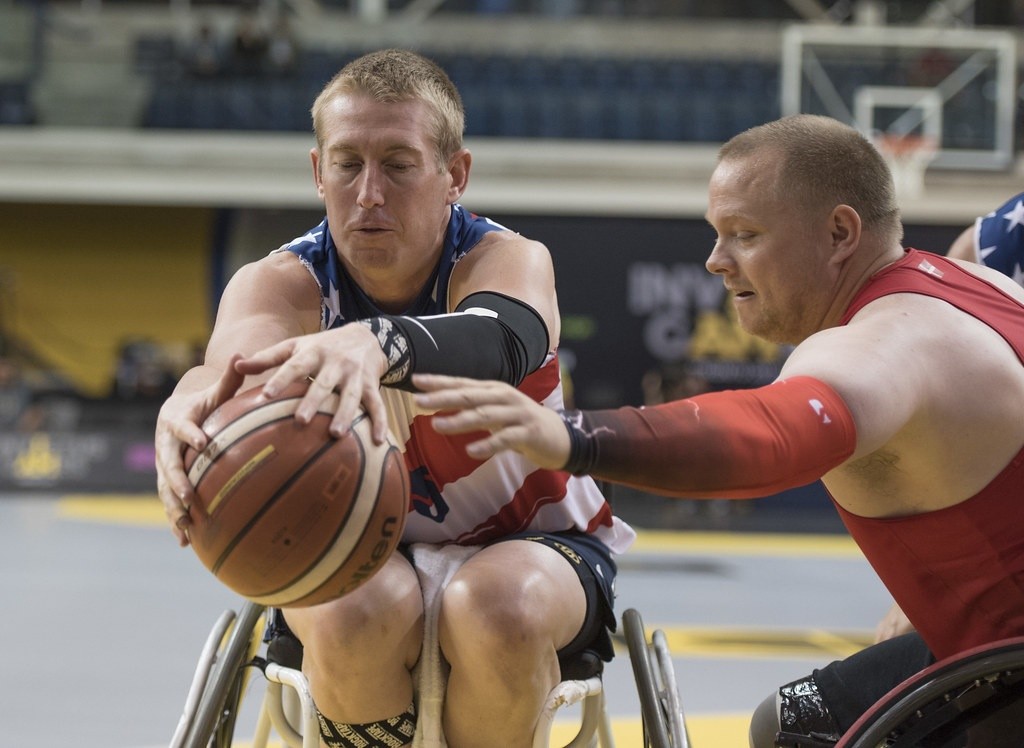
[832, 638, 1024, 748]
[163, 601, 695, 748]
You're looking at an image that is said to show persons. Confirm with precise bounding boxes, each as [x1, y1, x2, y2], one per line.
[155, 50, 637, 748]
[945, 190, 1024, 278]
[187, 25, 274, 87]
[413, 115, 1024, 748]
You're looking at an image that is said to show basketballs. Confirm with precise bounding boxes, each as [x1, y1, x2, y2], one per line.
[169, 368, 416, 611]
